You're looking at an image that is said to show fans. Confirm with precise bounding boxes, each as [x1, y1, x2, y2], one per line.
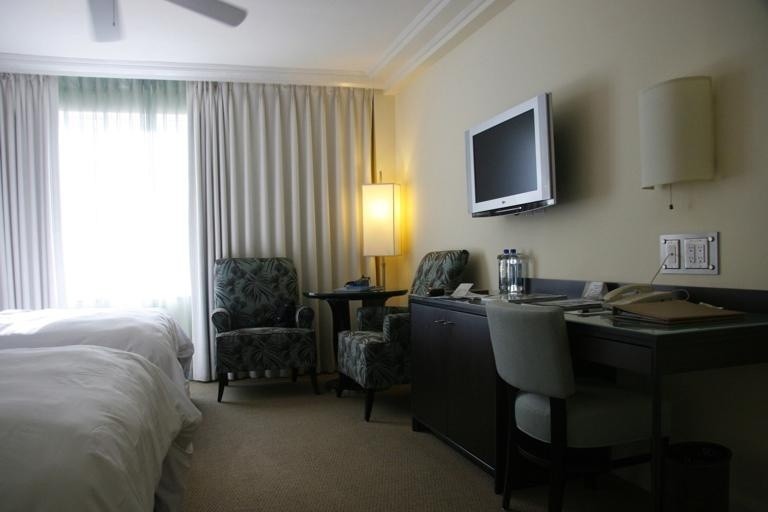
[86, 1, 250, 44]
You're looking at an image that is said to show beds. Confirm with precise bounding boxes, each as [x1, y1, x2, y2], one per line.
[0, 341, 204, 512]
[0, 306, 195, 394]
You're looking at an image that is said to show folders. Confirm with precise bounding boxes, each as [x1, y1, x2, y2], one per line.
[614, 300, 744, 323]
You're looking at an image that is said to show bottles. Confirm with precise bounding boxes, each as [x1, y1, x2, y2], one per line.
[499, 250, 508, 294]
[507, 249, 523, 293]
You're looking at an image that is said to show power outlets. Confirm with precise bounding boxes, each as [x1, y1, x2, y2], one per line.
[659, 232, 720, 275]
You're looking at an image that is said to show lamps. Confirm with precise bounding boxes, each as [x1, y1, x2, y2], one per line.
[636, 75, 717, 210]
[361, 181, 402, 290]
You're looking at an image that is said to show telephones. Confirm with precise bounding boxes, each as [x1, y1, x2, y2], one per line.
[602, 283, 673, 311]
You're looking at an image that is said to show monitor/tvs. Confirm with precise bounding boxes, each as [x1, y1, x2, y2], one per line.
[469, 92, 557, 219]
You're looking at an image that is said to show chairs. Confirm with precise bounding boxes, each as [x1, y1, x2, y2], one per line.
[211, 255, 320, 403]
[485, 300, 670, 512]
[335, 250, 469, 422]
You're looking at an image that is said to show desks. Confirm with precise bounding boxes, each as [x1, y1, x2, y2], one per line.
[401, 277, 591, 493]
[304, 290, 409, 392]
